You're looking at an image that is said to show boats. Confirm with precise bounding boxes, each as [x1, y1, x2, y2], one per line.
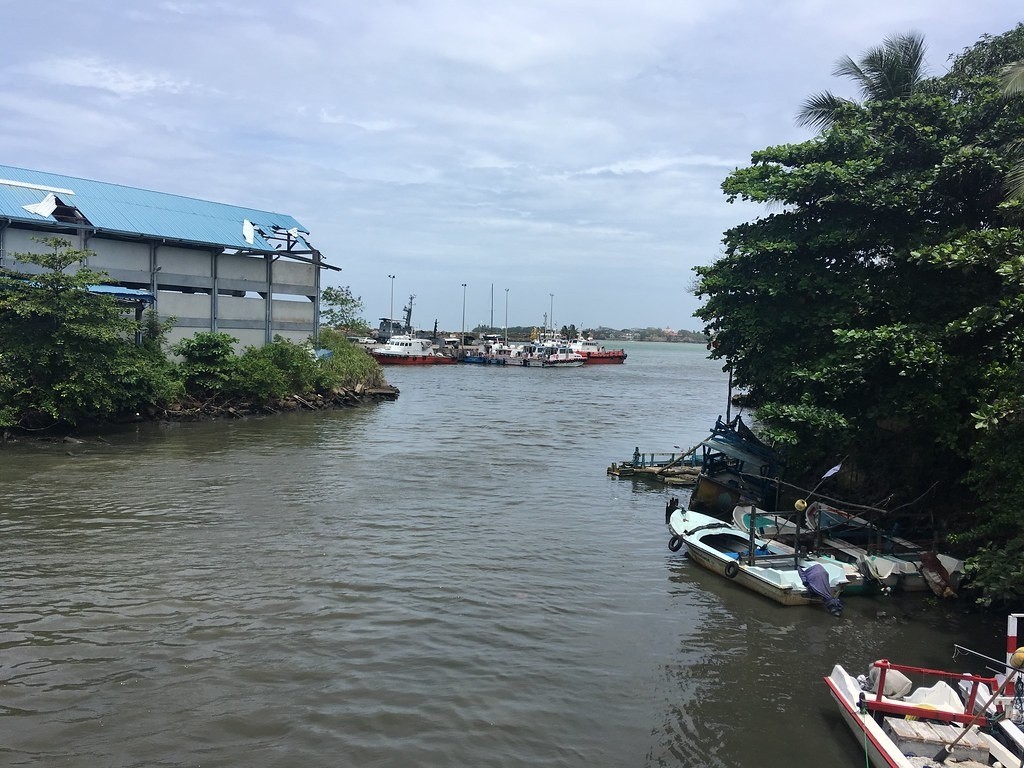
[439, 329, 628, 368]
[957, 612, 1024, 753]
[732, 501, 903, 597]
[806, 500, 960, 601]
[669, 503, 852, 618]
[606, 445, 721, 476]
[822, 657, 1022, 768]
[369, 326, 459, 365]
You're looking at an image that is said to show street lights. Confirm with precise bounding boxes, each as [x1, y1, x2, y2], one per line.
[503, 288, 510, 345]
[462, 283, 467, 351]
[387, 275, 396, 338]
[548, 293, 554, 332]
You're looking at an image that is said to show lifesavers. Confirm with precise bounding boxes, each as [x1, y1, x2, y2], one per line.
[724, 560, 739, 577]
[666, 536, 683, 551]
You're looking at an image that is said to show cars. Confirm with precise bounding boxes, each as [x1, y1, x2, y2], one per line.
[359, 336, 378, 344]
